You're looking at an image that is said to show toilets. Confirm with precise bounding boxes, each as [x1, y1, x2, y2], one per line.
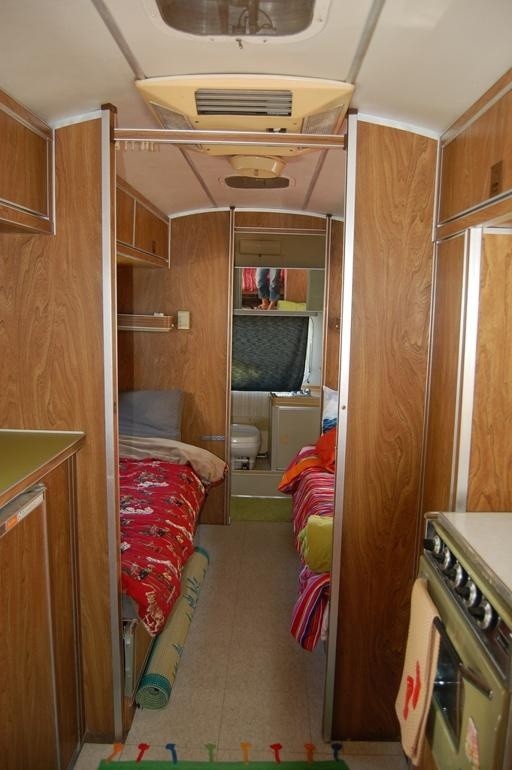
[230, 422, 261, 469]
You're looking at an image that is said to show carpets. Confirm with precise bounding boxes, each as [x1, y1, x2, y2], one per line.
[97, 741, 350, 770]
[228, 497, 294, 522]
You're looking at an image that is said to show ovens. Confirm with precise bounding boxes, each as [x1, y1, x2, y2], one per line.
[416, 553, 510, 770]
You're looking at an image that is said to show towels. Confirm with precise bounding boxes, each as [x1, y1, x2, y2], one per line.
[395, 575, 441, 770]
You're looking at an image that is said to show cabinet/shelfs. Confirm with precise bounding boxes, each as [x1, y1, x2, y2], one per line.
[0, 89, 173, 770]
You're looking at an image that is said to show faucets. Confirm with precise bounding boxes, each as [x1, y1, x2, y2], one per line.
[297, 388, 311, 395]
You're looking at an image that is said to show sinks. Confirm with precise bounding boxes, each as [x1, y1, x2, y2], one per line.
[270, 391, 309, 397]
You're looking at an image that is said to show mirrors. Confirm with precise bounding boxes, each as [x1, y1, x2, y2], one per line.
[233, 267, 308, 311]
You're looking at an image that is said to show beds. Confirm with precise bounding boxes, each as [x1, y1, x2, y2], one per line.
[119, 436, 206, 696]
[279, 427, 338, 654]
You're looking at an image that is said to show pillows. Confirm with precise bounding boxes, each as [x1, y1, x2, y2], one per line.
[118, 389, 185, 441]
[322, 386, 339, 433]
